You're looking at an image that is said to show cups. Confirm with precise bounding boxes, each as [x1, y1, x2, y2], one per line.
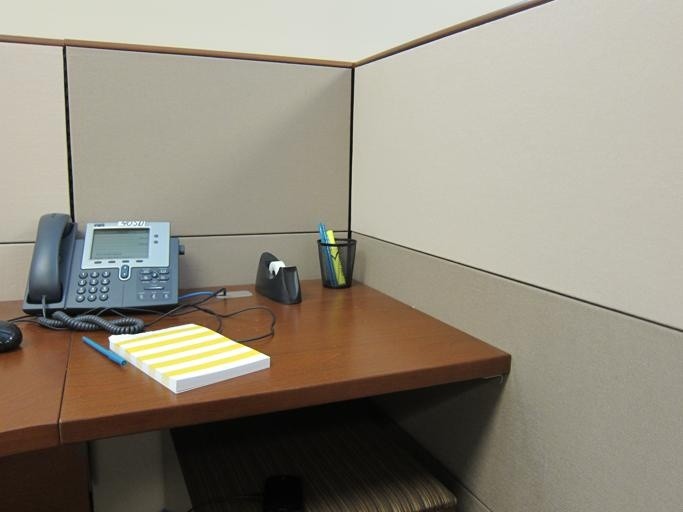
[318, 238, 356, 289]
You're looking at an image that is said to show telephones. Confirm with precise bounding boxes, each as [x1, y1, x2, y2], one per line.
[21, 213, 184, 315]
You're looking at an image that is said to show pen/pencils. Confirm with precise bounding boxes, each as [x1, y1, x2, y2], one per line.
[81, 336, 127, 366]
[319, 224, 346, 287]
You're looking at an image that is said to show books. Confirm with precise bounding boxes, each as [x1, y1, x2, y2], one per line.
[106, 322, 271, 396]
